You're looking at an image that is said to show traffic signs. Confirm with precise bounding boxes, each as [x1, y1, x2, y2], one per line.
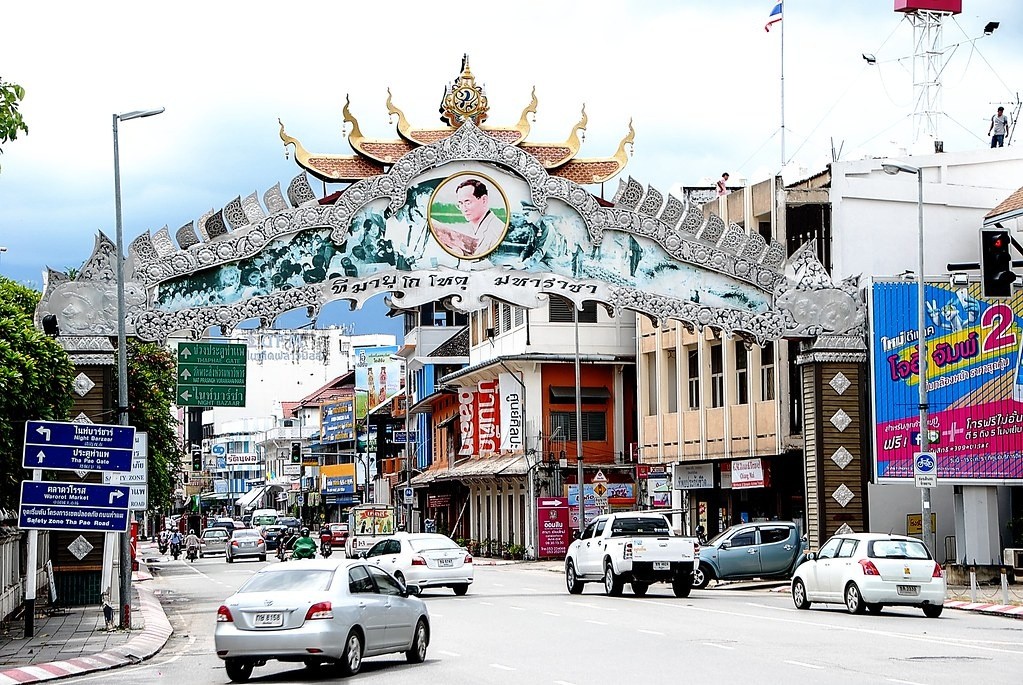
[176, 344, 247, 409]
[18, 419, 135, 534]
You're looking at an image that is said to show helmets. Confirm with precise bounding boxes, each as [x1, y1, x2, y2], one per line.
[288, 527, 294, 534]
[280, 528, 288, 533]
[324, 524, 330, 529]
[300, 527, 310, 537]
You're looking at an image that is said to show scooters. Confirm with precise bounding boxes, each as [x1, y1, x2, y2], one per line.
[276, 535, 331, 562]
[170, 543, 181, 559]
[159, 541, 168, 554]
[187, 546, 197, 562]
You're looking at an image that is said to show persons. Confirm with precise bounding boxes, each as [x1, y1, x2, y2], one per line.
[158, 527, 202, 558]
[444, 179, 506, 258]
[274, 525, 333, 562]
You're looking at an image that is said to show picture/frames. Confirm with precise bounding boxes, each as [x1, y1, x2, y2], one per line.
[428, 170, 510, 259]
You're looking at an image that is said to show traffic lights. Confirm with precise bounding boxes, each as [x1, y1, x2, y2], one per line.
[981, 227, 1016, 299]
[290, 441, 302, 464]
[192, 451, 203, 471]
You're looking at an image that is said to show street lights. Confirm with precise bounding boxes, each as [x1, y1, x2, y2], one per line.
[113, 105, 165, 621]
[881, 162, 936, 558]
[289, 416, 302, 525]
[354, 387, 369, 503]
[391, 355, 412, 532]
[225, 437, 234, 518]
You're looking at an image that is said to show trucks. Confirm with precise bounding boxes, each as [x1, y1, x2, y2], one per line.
[345, 505, 396, 557]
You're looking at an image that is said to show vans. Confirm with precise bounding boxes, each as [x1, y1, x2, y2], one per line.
[252, 509, 278, 529]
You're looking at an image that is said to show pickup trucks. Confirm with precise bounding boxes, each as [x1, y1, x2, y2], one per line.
[564, 512, 701, 598]
[690, 521, 810, 589]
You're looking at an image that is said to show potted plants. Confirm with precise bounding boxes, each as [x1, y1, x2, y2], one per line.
[458, 537, 528, 560]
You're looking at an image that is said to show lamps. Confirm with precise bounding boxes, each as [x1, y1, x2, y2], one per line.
[899, 272, 915, 284]
[943, 274, 969, 287]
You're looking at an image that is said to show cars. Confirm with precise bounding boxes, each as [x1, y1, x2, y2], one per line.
[163, 514, 300, 563]
[791, 533, 945, 618]
[329, 522, 350, 545]
[215, 556, 431, 682]
[361, 531, 475, 597]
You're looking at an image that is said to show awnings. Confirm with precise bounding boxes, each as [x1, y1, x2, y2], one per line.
[395, 453, 527, 490]
[235, 485, 271, 507]
[201, 493, 241, 500]
